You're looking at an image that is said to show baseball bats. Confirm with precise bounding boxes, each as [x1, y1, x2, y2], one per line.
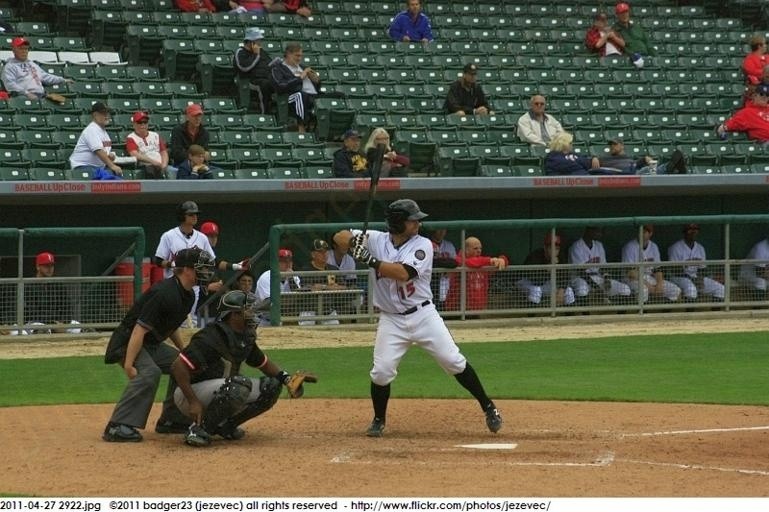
[363, 143, 386, 235]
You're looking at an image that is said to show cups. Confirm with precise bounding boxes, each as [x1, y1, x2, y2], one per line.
[648, 160, 657, 174]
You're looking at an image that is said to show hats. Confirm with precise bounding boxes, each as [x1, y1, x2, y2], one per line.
[345, 129, 362, 139]
[244, 28, 264, 40]
[279, 248, 294, 260]
[616, 3, 629, 13]
[36, 252, 54, 264]
[186, 104, 203, 115]
[464, 63, 478, 74]
[177, 201, 201, 222]
[756, 85, 769, 94]
[133, 112, 148, 122]
[544, 234, 561, 249]
[12, 38, 30, 48]
[92, 103, 109, 113]
[201, 223, 219, 235]
[312, 240, 329, 251]
[607, 138, 624, 144]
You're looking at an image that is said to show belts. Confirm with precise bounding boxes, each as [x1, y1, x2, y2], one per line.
[398, 300, 430, 315]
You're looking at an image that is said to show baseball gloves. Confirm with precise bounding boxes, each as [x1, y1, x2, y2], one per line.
[288, 370, 318, 398]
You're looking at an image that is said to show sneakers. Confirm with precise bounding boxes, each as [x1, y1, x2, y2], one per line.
[185, 424, 207, 446]
[483, 400, 502, 432]
[210, 428, 244, 440]
[103, 422, 141, 442]
[666, 150, 687, 174]
[367, 417, 385, 436]
[155, 417, 188, 433]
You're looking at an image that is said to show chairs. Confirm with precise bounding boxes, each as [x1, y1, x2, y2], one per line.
[1, 0, 769, 183]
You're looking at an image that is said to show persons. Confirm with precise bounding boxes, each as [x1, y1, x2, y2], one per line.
[621, 224, 681, 314]
[23, 251, 70, 323]
[667, 224, 725, 312]
[517, 235, 574, 316]
[168, 289, 317, 448]
[2, 35, 73, 101]
[334, 200, 502, 437]
[567, 229, 631, 315]
[738, 238, 769, 309]
[102, 246, 217, 443]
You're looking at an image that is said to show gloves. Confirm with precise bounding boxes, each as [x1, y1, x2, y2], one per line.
[232, 257, 248, 271]
[352, 246, 377, 265]
[351, 233, 369, 246]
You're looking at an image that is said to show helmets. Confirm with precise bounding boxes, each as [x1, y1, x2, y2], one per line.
[172, 245, 216, 296]
[216, 290, 263, 346]
[386, 199, 428, 234]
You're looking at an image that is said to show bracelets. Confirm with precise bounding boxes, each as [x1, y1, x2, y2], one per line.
[277, 371, 291, 385]
